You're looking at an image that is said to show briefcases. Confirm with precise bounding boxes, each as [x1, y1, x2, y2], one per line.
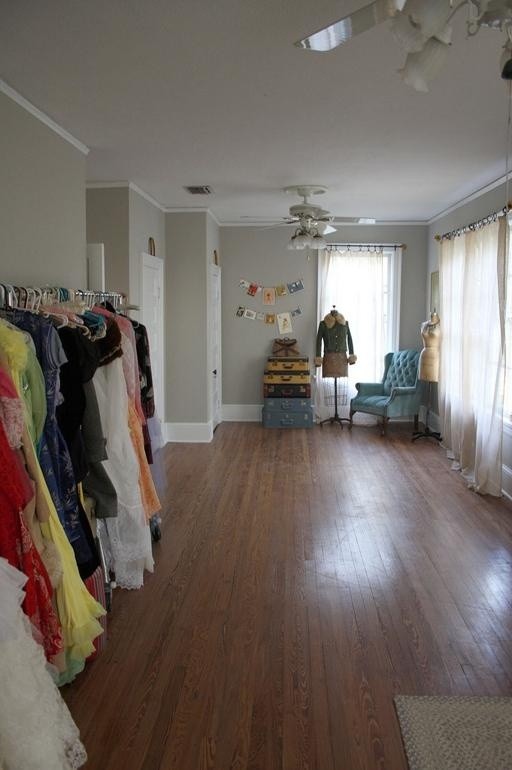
[262, 356, 313, 428]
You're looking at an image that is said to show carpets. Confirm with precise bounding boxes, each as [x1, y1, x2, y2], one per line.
[391, 694, 512, 770]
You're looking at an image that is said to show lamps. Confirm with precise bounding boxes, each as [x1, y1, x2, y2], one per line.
[393, 0, 512, 96]
[283, 230, 338, 251]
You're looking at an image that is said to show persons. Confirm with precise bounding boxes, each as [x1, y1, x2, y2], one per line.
[315, 311, 356, 379]
[417, 313, 441, 383]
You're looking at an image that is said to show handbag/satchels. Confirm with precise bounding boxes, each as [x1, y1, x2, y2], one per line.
[274, 338, 300, 357]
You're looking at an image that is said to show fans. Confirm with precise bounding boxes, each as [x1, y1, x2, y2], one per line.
[239, 185, 377, 234]
[294, 0, 406, 55]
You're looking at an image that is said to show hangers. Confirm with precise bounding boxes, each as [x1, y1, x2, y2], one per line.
[1, 282, 141, 343]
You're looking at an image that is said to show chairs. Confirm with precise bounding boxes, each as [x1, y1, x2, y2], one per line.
[348, 349, 430, 437]
